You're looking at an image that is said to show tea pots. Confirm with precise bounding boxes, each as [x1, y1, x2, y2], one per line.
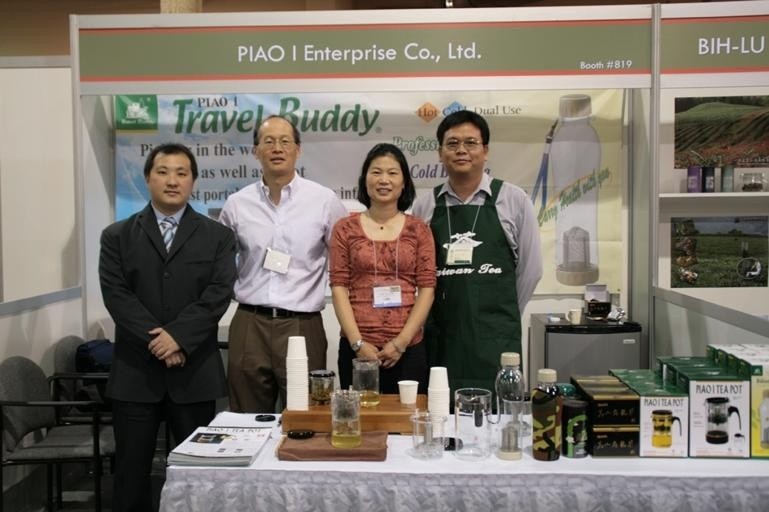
[652, 409, 683, 449]
[703, 398, 742, 445]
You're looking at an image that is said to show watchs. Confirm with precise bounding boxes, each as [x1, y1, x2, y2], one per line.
[351, 338, 366, 352]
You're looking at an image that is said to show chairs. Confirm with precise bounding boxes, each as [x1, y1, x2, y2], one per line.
[46, 338, 115, 512]
[0, 357, 108, 511]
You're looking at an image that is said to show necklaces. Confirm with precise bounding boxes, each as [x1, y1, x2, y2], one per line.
[367, 210, 399, 230]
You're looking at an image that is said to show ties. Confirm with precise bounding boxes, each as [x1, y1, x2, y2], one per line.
[161, 216, 178, 252]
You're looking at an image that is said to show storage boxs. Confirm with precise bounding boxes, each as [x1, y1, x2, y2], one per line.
[706, 343, 769, 459]
[609, 369, 688, 455]
[655, 357, 750, 457]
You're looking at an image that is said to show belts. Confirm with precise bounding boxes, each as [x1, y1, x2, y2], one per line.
[237, 302, 305, 317]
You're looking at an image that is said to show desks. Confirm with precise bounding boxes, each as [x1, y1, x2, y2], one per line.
[159, 413, 769, 512]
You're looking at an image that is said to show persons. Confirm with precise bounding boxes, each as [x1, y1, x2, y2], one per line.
[410, 110, 545, 414]
[98, 142, 236, 512]
[327, 142, 436, 394]
[217, 114, 350, 413]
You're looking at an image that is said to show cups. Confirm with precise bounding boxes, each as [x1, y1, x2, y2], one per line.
[398, 380, 420, 404]
[331, 390, 362, 448]
[454, 388, 492, 460]
[285, 335, 309, 412]
[565, 308, 582, 324]
[427, 366, 450, 420]
[353, 358, 381, 407]
[310, 369, 336, 405]
[410, 412, 449, 459]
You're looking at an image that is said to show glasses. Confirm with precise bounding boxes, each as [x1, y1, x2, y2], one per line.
[440, 139, 483, 149]
[260, 137, 297, 149]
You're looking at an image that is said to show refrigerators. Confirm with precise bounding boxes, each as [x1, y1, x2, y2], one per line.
[530, 312, 640, 382]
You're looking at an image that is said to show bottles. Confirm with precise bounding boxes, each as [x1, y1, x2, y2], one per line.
[531, 368, 559, 463]
[495, 352, 528, 459]
[758, 388, 768, 451]
[686, 164, 765, 192]
[556, 382, 576, 396]
[551, 94, 602, 285]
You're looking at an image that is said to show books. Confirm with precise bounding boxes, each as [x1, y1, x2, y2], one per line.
[167, 425, 273, 467]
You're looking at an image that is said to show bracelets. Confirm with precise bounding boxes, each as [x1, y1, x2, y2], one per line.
[392, 338, 406, 354]
